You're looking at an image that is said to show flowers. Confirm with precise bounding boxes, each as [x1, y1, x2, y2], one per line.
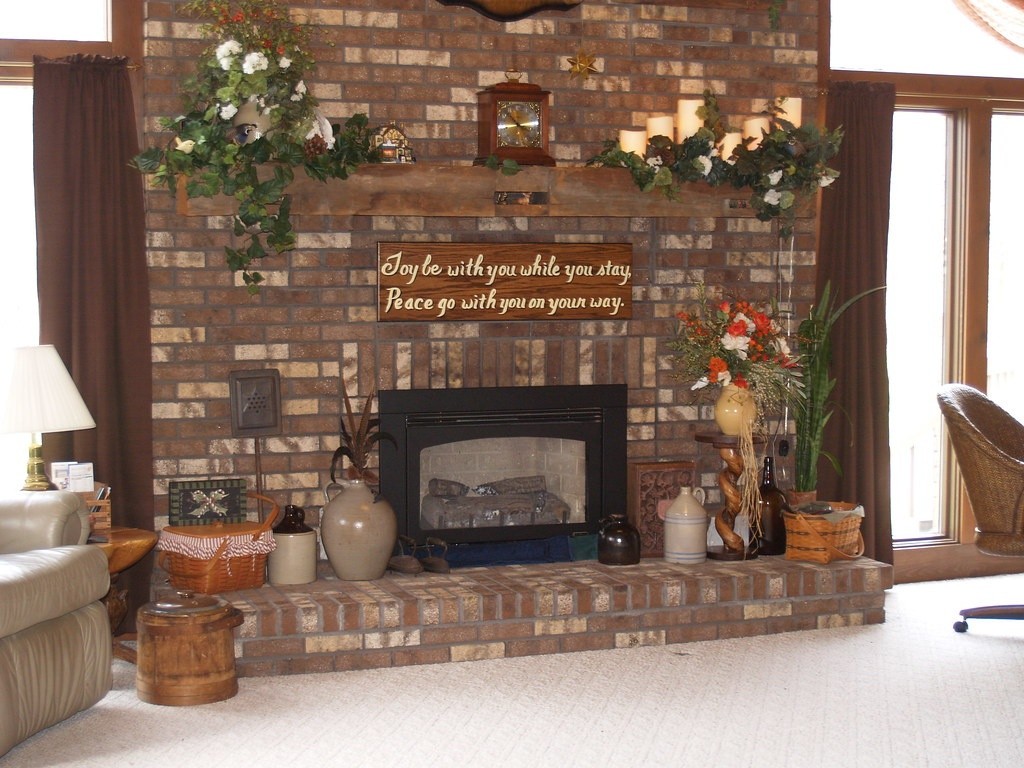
[584, 88, 846, 242]
[663, 268, 808, 426]
[127, 0, 385, 299]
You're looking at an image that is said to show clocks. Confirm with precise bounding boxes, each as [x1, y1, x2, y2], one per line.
[473, 69, 556, 168]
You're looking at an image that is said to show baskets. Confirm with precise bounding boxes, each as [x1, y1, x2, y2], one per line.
[157, 492, 279, 595]
[779, 500, 865, 563]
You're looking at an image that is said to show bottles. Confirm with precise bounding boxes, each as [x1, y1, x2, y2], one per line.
[665, 488, 708, 564]
[270, 505, 318, 585]
[757, 457, 786, 555]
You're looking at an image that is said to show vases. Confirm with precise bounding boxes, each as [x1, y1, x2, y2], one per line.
[231, 94, 269, 162]
[320, 478, 397, 581]
[714, 379, 758, 435]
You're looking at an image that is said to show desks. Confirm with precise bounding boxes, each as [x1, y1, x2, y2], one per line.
[88, 527, 159, 665]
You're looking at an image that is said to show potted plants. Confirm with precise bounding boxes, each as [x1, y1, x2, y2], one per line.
[785, 278, 891, 507]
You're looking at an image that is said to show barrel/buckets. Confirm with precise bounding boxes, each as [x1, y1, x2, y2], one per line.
[138, 593, 243, 706]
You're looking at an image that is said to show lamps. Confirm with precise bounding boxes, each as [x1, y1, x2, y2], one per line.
[0, 345, 96, 489]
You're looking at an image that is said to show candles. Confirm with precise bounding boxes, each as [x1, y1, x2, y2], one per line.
[715, 133, 745, 166]
[677, 98, 705, 145]
[745, 118, 769, 152]
[619, 129, 647, 167]
[775, 98, 802, 133]
[645, 116, 673, 149]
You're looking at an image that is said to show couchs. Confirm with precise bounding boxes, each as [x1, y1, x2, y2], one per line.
[0, 491, 113, 758]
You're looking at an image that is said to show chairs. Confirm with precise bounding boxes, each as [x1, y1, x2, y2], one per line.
[937, 383, 1024, 632]
